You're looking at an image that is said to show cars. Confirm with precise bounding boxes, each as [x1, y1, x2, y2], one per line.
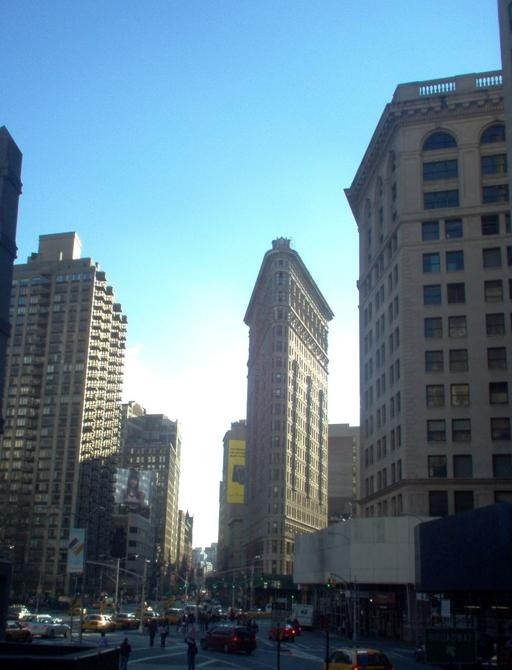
[268, 616, 302, 643]
[6, 603, 249, 640]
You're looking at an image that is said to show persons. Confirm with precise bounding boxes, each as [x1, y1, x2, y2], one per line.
[118, 467, 148, 504]
[148, 610, 260, 648]
[184, 632, 196, 670]
[97, 632, 111, 647]
[286, 616, 299, 626]
[119, 638, 131, 670]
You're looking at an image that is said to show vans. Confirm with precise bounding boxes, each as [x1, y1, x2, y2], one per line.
[199, 624, 257, 654]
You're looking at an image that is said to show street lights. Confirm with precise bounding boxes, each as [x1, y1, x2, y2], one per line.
[99, 553, 140, 604]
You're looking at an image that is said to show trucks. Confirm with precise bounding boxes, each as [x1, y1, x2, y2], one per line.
[286, 603, 314, 630]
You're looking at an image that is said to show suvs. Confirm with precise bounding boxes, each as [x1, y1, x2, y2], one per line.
[320, 647, 395, 670]
